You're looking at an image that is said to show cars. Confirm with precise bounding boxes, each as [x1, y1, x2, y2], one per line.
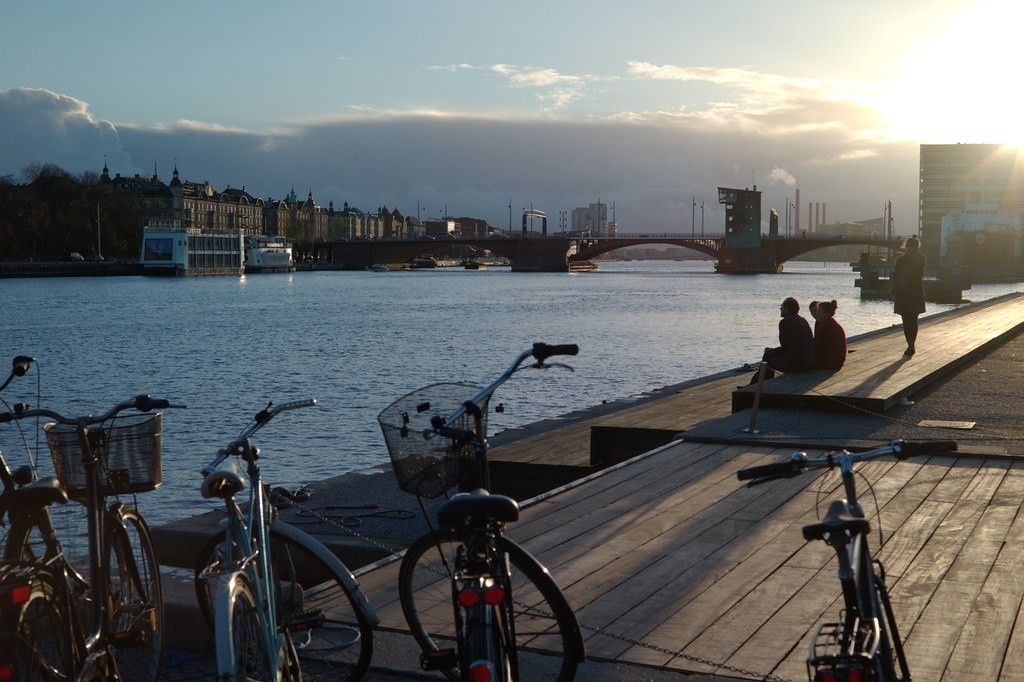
[65, 252, 85, 263]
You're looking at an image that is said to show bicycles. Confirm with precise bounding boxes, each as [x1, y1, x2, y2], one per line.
[737, 439, 960, 682]
[193, 396, 382, 682]
[376, 340, 585, 682]
[0, 354, 188, 682]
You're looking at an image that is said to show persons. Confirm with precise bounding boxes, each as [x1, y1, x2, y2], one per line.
[737, 297, 814, 390]
[813, 299, 847, 370]
[894, 234, 927, 354]
[809, 301, 822, 319]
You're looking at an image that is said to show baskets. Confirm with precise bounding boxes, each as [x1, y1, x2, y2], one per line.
[42, 410, 163, 502]
[378, 382, 491, 500]
